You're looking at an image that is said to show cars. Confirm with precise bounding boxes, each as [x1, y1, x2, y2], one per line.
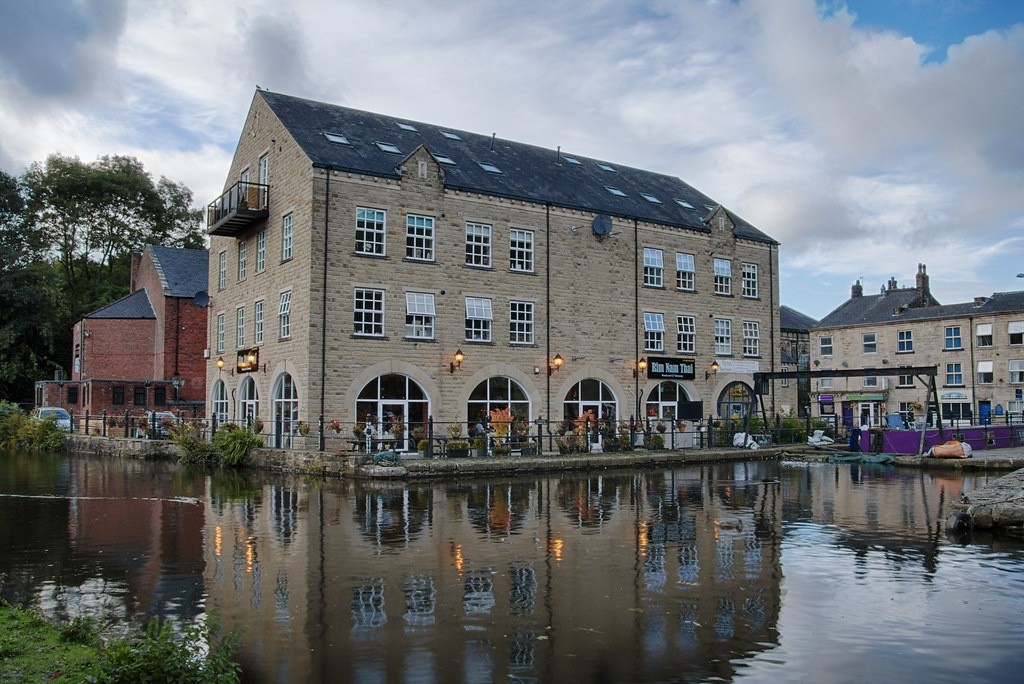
[32, 407, 75, 434]
[135, 410, 179, 437]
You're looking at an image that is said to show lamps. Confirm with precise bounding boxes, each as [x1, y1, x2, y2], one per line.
[450, 348, 464, 374]
[247, 351, 266, 373]
[705, 361, 720, 380]
[549, 352, 563, 376]
[217, 356, 233, 377]
[633, 357, 647, 379]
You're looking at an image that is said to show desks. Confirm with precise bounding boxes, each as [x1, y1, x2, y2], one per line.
[345, 436, 470, 456]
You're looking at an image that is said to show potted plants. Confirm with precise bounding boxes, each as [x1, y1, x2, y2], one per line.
[554, 436, 573, 454]
[643, 434, 665, 450]
[445, 422, 462, 437]
[907, 399, 922, 411]
[480, 405, 513, 456]
[519, 443, 538, 455]
[635, 422, 642, 432]
[556, 422, 566, 435]
[445, 441, 469, 458]
[676, 423, 686, 432]
[474, 435, 487, 456]
[417, 440, 429, 458]
[656, 421, 667, 433]
[573, 409, 596, 452]
[512, 407, 532, 435]
[604, 437, 622, 452]
[103, 414, 426, 449]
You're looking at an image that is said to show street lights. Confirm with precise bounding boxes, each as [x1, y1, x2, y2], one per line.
[58, 381, 63, 407]
[172, 376, 186, 416]
[143, 379, 151, 410]
